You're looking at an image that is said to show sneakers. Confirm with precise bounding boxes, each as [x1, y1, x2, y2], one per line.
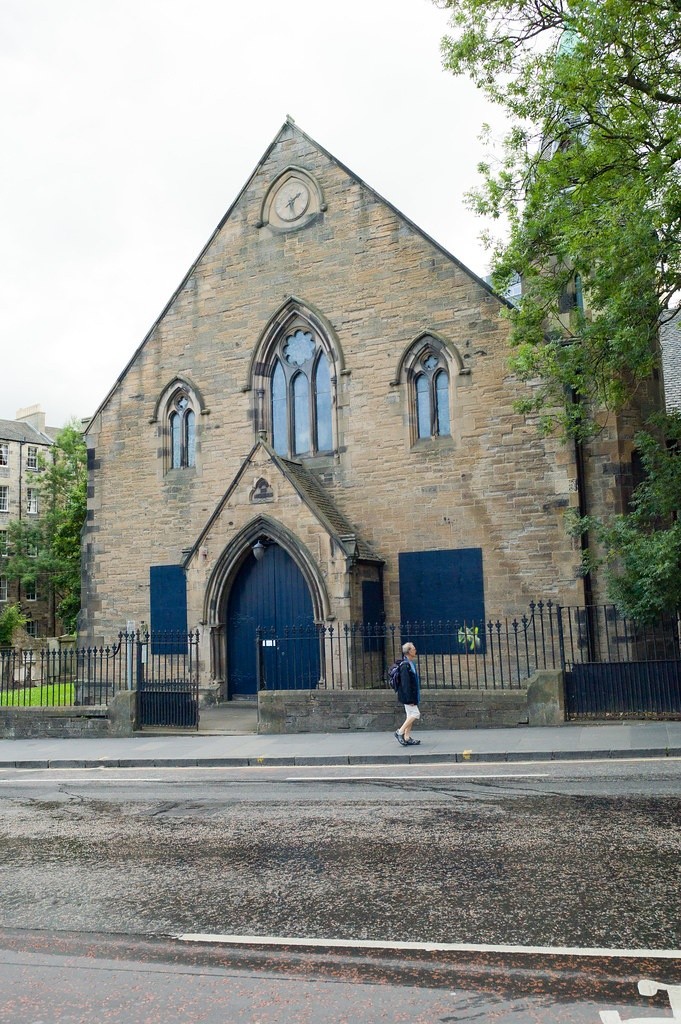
[405, 738, 420, 745]
[395, 728, 407, 746]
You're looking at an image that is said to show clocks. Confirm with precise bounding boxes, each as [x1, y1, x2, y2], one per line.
[255, 164, 327, 234]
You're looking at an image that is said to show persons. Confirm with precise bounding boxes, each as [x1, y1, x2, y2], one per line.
[393, 641, 422, 745]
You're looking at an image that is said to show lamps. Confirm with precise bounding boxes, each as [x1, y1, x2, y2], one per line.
[252, 540, 268, 561]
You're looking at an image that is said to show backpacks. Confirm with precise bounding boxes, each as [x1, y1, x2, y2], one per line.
[389, 662, 400, 691]
